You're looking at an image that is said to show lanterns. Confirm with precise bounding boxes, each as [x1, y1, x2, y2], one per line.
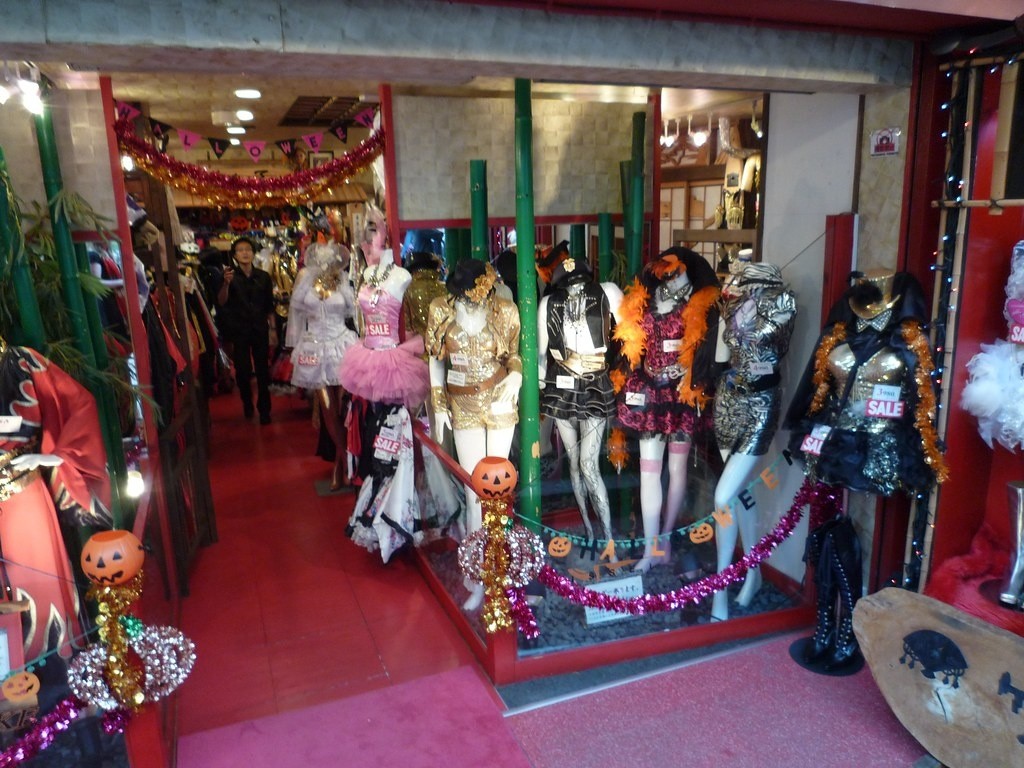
[471, 456, 517, 499]
[81, 530, 150, 586]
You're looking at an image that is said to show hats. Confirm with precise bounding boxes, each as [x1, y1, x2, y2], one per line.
[738, 262, 783, 292]
[651, 255, 686, 281]
[407, 252, 443, 273]
[445, 258, 497, 305]
[507, 230, 517, 248]
[845, 268, 900, 320]
[550, 257, 595, 292]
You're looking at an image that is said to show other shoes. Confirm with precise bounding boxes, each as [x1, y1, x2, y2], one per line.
[243, 403, 254, 421]
[257, 409, 271, 425]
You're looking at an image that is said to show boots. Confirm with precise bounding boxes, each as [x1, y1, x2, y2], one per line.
[802, 527, 838, 660]
[826, 514, 863, 667]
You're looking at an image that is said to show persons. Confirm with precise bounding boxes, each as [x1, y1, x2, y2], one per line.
[537, 254, 624, 577]
[338, 248, 429, 408]
[424, 259, 522, 612]
[285, 237, 355, 491]
[605, 246, 722, 573]
[0, 337, 110, 675]
[788, 268, 950, 670]
[710, 262, 797, 624]
[85, 242, 177, 430]
[215, 237, 278, 426]
[289, 147, 309, 173]
